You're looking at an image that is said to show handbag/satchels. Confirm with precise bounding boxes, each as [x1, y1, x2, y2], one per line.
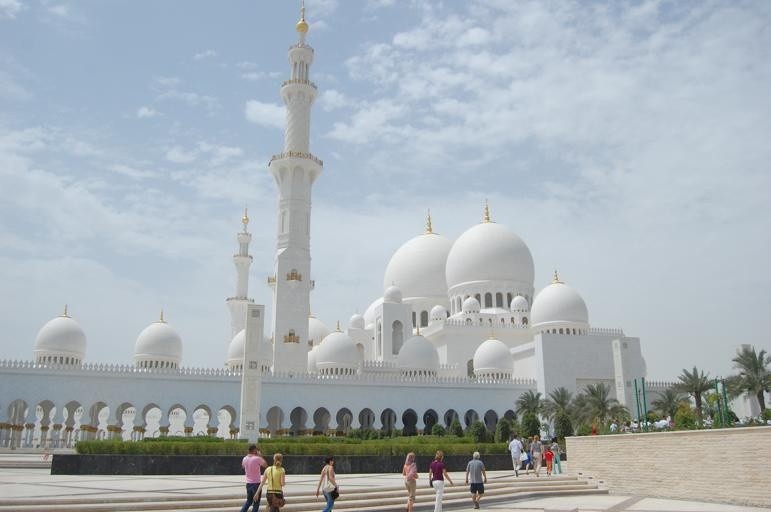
[331, 486, 340, 500]
[519, 450, 529, 462]
[271, 492, 286, 508]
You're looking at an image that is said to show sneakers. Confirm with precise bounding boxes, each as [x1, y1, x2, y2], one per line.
[473, 501, 480, 509]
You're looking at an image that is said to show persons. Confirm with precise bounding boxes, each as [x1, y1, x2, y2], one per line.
[401, 452, 420, 511]
[429, 450, 454, 512]
[509, 434, 565, 478]
[590, 413, 677, 435]
[240, 445, 267, 512]
[252, 453, 286, 511]
[465, 452, 488, 509]
[315, 456, 339, 512]
